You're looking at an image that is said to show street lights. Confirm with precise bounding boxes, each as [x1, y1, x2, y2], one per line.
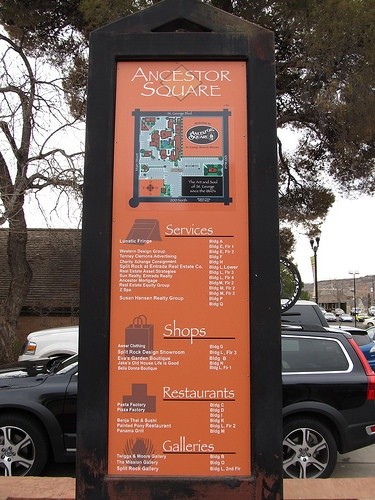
[349, 272, 362, 328]
[308, 228, 322, 304]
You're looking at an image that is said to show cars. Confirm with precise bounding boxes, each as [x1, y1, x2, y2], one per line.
[351, 308, 362, 315]
[368, 306, 375, 316]
[356, 313, 369, 322]
[363, 317, 375, 328]
[323, 313, 337, 321]
[366, 326, 375, 342]
[333, 327, 375, 368]
[339, 314, 353, 322]
[335, 309, 344, 315]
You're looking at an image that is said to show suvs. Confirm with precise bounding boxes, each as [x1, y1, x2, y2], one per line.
[1, 321, 375, 479]
[18, 299, 330, 370]
